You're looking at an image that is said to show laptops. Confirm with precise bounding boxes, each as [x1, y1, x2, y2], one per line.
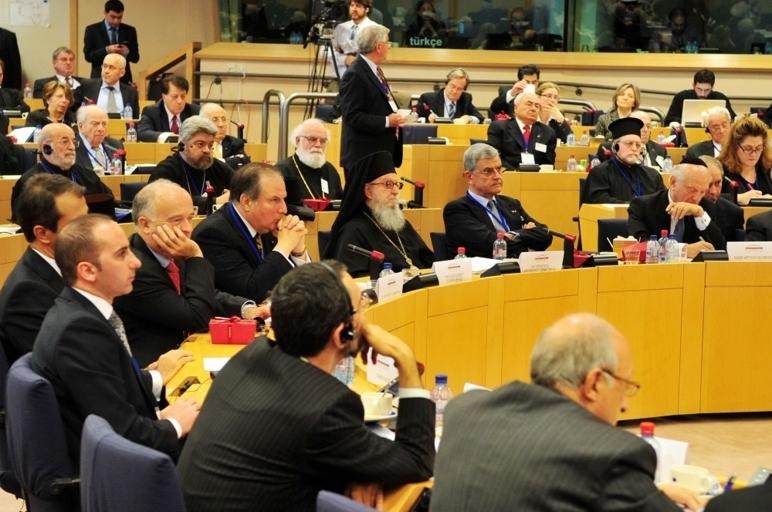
[681, 99, 727, 127]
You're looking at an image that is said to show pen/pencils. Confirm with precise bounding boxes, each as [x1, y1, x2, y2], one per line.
[723, 475, 736, 494]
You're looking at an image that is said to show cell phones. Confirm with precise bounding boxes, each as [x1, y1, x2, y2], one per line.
[118, 41, 128, 48]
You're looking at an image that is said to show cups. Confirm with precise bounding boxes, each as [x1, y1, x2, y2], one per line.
[670, 465, 713, 495]
[360, 389, 393, 415]
[484, 118, 491, 124]
[623, 247, 640, 265]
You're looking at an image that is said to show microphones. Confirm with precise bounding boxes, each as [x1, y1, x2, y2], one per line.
[541, 227, 576, 268]
[400, 175, 425, 210]
[229, 119, 245, 141]
[671, 123, 681, 148]
[104, 144, 127, 175]
[84, 96, 93, 103]
[722, 174, 740, 192]
[204, 179, 216, 216]
[346, 244, 385, 286]
[601, 146, 611, 156]
[582, 107, 596, 126]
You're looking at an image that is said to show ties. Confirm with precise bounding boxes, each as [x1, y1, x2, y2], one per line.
[107, 86, 117, 113]
[523, 124, 531, 145]
[108, 27, 117, 44]
[448, 101, 454, 119]
[377, 66, 391, 94]
[92, 146, 106, 170]
[254, 232, 265, 260]
[166, 261, 182, 297]
[64, 76, 72, 89]
[170, 115, 179, 134]
[674, 214, 686, 242]
[349, 25, 357, 40]
[487, 200, 505, 234]
[109, 310, 133, 359]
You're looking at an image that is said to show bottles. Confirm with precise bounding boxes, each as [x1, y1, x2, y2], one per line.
[334, 356, 354, 386]
[568, 154, 577, 171]
[127, 122, 138, 143]
[110, 153, 123, 175]
[24, 82, 32, 98]
[591, 155, 600, 169]
[686, 40, 698, 53]
[657, 130, 665, 142]
[379, 261, 395, 277]
[409, 107, 418, 122]
[566, 130, 576, 145]
[493, 232, 508, 259]
[646, 229, 679, 262]
[579, 130, 589, 145]
[428, 375, 453, 437]
[635, 421, 665, 486]
[454, 246, 468, 259]
[662, 155, 673, 172]
[124, 102, 133, 119]
[33, 125, 42, 144]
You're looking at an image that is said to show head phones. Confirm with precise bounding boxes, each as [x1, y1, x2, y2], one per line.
[613, 143, 621, 151]
[311, 261, 356, 346]
[32, 145, 52, 155]
[170, 142, 185, 154]
[364, 5, 373, 15]
[704, 126, 710, 134]
[444, 79, 469, 90]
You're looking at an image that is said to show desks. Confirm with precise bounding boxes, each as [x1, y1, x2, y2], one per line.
[1, 84, 771, 511]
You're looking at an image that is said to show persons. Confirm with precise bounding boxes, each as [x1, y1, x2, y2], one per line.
[424, 311, 706, 510]
[169, 263, 437, 511]
[81, 0, 142, 86]
[2, 28, 24, 95]
[30, 209, 201, 473]
[0, 169, 196, 501]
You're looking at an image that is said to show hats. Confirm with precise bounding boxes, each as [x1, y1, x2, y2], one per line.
[607, 117, 645, 140]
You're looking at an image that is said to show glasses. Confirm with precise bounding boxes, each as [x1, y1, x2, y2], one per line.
[541, 93, 558, 99]
[469, 166, 503, 175]
[738, 142, 764, 155]
[370, 181, 404, 189]
[599, 367, 640, 396]
[299, 134, 326, 146]
[49, 138, 79, 147]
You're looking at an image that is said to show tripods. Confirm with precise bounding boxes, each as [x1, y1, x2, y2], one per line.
[301, 40, 341, 120]
